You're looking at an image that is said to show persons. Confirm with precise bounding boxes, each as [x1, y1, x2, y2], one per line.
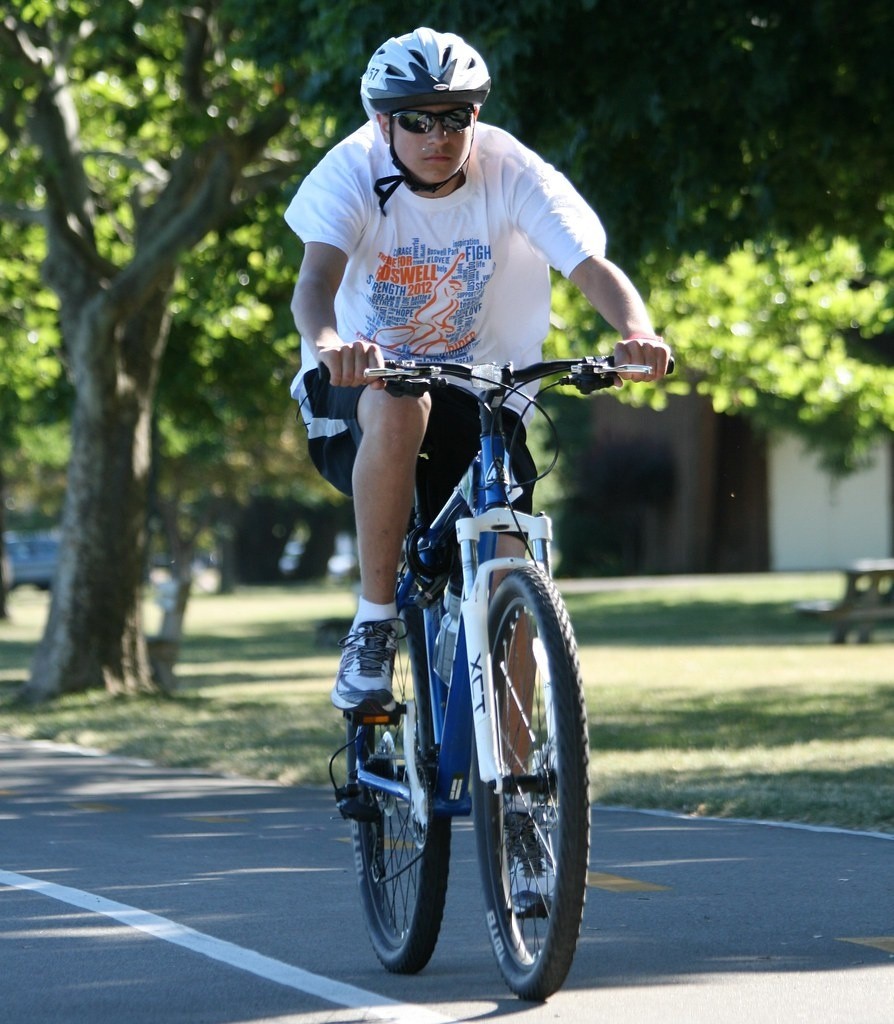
[287, 27, 670, 916]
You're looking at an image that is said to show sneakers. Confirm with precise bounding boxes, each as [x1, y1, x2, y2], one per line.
[330, 616, 410, 715]
[501, 802, 556, 919]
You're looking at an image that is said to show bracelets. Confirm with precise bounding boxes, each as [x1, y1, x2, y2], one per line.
[625, 333, 663, 341]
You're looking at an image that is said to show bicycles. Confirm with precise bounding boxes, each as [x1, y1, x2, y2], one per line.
[318, 355, 676, 1001]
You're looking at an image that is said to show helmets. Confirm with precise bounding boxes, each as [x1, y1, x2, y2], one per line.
[359, 27, 493, 108]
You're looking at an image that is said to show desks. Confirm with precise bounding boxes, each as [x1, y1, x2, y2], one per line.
[830, 555, 894, 646]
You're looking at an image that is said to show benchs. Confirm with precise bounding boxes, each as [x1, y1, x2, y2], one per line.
[798, 601, 893, 624]
[0, 536, 64, 619]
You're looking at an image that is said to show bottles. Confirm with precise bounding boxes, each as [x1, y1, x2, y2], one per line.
[432, 572, 464, 687]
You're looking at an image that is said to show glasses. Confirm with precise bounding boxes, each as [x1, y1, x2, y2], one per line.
[392, 106, 476, 133]
